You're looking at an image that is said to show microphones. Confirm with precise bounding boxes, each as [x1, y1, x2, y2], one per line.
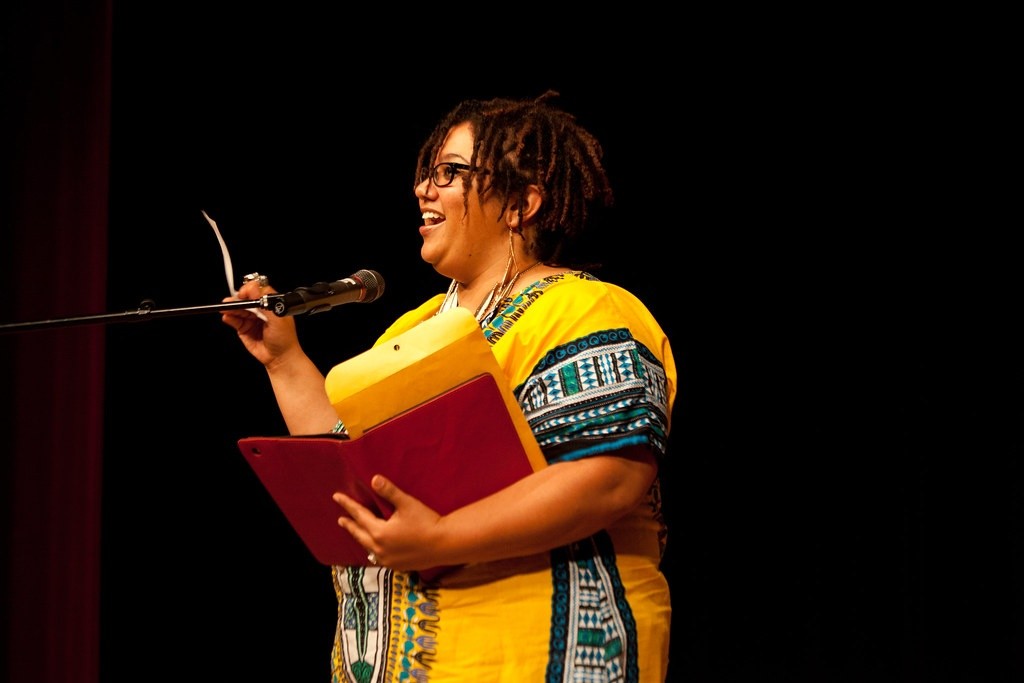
[272, 269, 385, 317]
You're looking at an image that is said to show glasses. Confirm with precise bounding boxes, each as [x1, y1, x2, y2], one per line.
[419, 161, 499, 188]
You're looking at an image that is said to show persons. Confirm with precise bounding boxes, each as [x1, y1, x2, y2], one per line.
[222, 97, 678, 683]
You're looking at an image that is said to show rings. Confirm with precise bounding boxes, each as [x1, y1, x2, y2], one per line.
[242, 271, 268, 286]
[367, 552, 376, 564]
